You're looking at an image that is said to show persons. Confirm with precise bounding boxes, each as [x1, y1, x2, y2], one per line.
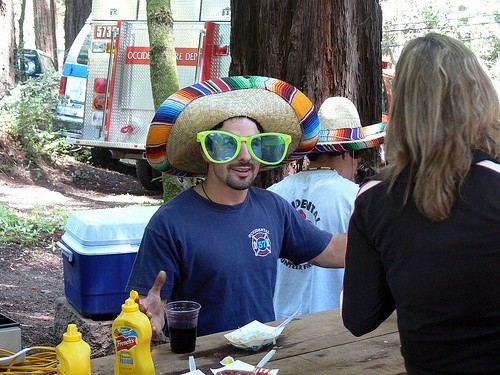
[265, 95, 388, 320]
[340, 32, 499, 375]
[127, 77, 348, 337]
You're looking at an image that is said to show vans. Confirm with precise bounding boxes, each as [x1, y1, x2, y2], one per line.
[56, 12, 94, 125]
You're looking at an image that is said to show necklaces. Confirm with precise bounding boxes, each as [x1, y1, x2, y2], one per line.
[201, 181, 214, 203]
[302, 165, 338, 174]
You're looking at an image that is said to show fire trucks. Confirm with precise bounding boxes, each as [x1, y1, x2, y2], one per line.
[72, 0, 397, 196]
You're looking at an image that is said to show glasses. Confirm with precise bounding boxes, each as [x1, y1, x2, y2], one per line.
[195, 131, 292, 165]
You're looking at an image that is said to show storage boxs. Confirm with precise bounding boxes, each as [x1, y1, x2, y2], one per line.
[54, 205, 160, 320]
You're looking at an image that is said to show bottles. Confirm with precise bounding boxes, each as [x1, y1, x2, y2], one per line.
[56, 324, 92, 375]
[112, 290, 156, 375]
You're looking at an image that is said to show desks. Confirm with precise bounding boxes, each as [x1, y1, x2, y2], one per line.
[91, 309, 407, 375]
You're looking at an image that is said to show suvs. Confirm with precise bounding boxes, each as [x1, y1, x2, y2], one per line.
[15, 48, 56, 84]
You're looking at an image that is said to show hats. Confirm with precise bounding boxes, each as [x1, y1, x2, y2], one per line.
[146, 75, 321, 176]
[310, 96, 388, 153]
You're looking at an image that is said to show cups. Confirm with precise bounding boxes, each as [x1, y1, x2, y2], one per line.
[163, 301, 201, 355]
